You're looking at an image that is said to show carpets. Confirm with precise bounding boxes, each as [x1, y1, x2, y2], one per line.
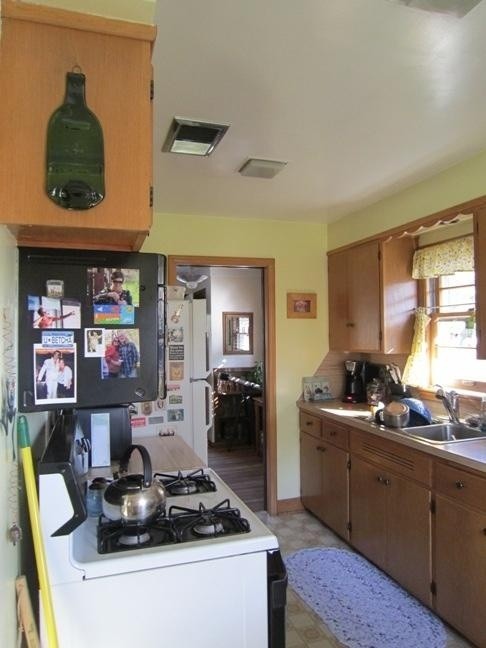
[283, 547, 457, 648]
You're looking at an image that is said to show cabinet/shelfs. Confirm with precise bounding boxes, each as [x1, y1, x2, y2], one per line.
[299, 407, 351, 544]
[328, 239, 417, 355]
[350, 425, 432, 611]
[0, 0, 154, 255]
[431, 455, 486, 648]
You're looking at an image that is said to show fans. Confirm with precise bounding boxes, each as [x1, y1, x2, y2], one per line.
[176, 274, 208, 302]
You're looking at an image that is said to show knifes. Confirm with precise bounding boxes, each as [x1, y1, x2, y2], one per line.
[384, 363, 403, 384]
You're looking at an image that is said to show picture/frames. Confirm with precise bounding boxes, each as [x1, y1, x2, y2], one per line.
[286, 292, 317, 319]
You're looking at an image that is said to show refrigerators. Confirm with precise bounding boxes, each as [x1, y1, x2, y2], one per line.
[131, 299, 213, 468]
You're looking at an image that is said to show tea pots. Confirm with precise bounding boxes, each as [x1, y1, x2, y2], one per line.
[102, 444, 167, 525]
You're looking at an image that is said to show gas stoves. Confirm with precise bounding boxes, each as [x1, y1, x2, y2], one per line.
[72, 468, 275, 562]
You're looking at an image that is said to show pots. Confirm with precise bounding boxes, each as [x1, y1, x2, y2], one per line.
[383, 402, 409, 427]
[400, 397, 432, 425]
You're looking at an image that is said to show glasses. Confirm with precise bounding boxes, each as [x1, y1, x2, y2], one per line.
[112, 281, 123, 284]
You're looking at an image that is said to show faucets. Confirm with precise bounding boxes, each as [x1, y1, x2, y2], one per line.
[436, 389, 460, 424]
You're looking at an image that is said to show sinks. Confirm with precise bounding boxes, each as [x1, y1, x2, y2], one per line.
[401, 422, 486, 444]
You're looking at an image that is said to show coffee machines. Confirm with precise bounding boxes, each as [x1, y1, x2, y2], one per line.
[343, 360, 374, 403]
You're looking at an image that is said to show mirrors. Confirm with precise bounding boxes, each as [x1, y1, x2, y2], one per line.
[222, 311, 254, 355]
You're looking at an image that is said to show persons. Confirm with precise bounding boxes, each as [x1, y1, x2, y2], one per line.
[94, 271, 132, 305]
[313, 384, 333, 400]
[89, 332, 101, 353]
[33, 304, 75, 328]
[105, 333, 139, 377]
[37, 351, 73, 399]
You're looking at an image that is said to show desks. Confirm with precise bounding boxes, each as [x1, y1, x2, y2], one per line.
[252, 397, 264, 459]
[82, 431, 207, 482]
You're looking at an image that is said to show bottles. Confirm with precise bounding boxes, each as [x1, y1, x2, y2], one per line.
[43, 71, 105, 208]
[366, 379, 387, 407]
[171, 304, 184, 322]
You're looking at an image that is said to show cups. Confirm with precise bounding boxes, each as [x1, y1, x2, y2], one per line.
[87, 476, 112, 517]
